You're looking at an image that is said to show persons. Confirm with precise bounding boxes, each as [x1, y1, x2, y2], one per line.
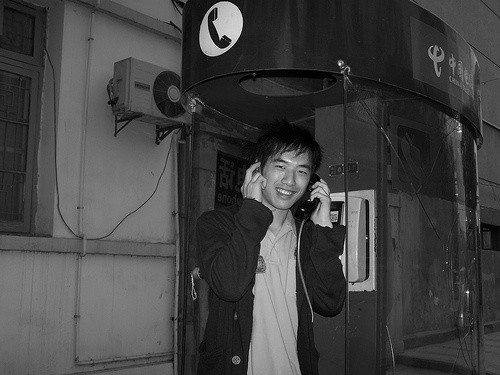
[194, 122, 347, 375]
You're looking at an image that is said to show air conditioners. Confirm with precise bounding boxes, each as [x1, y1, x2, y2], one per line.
[107, 56, 193, 129]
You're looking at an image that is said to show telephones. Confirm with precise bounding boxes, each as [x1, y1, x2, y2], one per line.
[298, 173, 328, 221]
